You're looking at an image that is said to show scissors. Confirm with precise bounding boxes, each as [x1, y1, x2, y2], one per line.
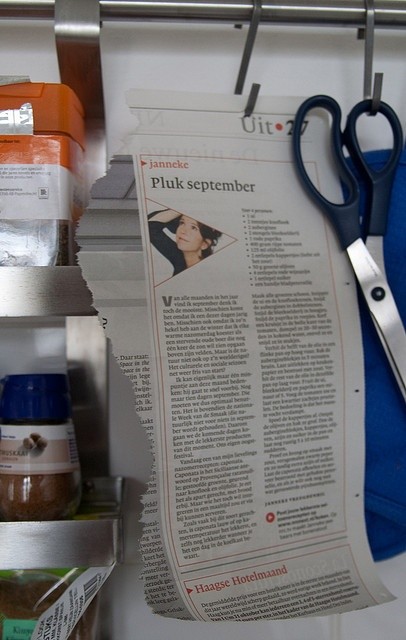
[291, 97, 406, 396]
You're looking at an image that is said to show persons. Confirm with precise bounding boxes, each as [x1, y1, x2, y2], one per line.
[148, 208, 223, 278]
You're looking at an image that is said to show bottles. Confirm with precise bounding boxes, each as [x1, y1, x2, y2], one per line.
[0, 373, 87, 522]
